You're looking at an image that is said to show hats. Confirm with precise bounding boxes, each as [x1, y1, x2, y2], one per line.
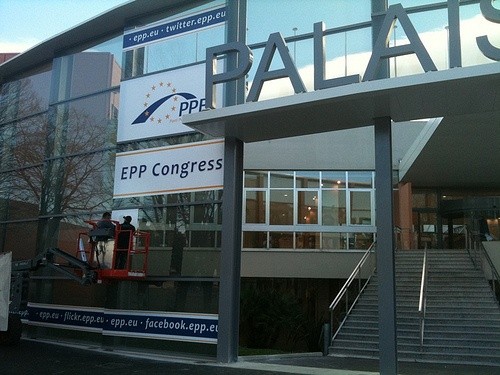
[138, 218, 147, 222]
[123, 216, 132, 221]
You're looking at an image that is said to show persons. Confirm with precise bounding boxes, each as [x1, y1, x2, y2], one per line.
[114, 215, 136, 270]
[479, 216, 490, 242]
[93, 213, 116, 270]
